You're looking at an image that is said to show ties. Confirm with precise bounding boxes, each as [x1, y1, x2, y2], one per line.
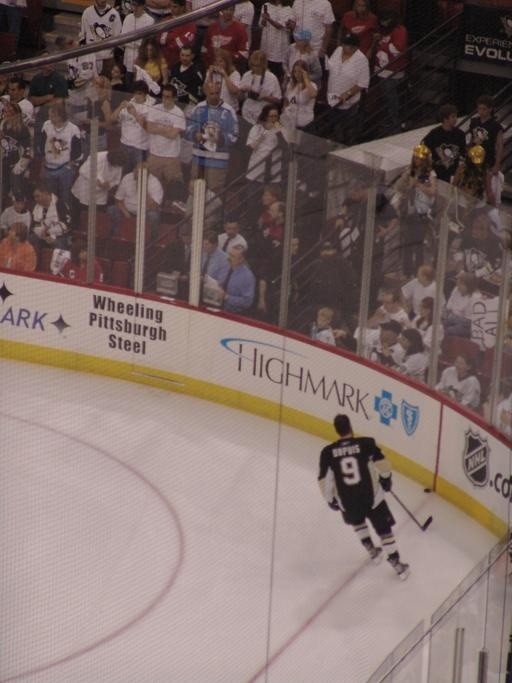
[222, 268, 232, 288]
[223, 238, 231, 252]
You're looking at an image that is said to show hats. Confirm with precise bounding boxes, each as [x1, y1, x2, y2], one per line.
[379, 319, 402, 333]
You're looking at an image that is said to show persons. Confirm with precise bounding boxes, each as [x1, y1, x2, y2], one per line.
[318, 412, 410, 574]
[0, 0, 511, 444]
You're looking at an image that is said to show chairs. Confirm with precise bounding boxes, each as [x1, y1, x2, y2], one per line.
[26, 112, 510, 420]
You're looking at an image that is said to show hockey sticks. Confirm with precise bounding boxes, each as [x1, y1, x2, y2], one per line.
[390, 490, 432, 532]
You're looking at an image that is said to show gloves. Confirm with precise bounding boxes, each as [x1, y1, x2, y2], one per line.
[328, 496, 339, 511]
[378, 473, 393, 492]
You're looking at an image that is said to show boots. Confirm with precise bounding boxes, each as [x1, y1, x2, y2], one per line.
[368, 546, 382, 559]
[387, 556, 408, 575]
